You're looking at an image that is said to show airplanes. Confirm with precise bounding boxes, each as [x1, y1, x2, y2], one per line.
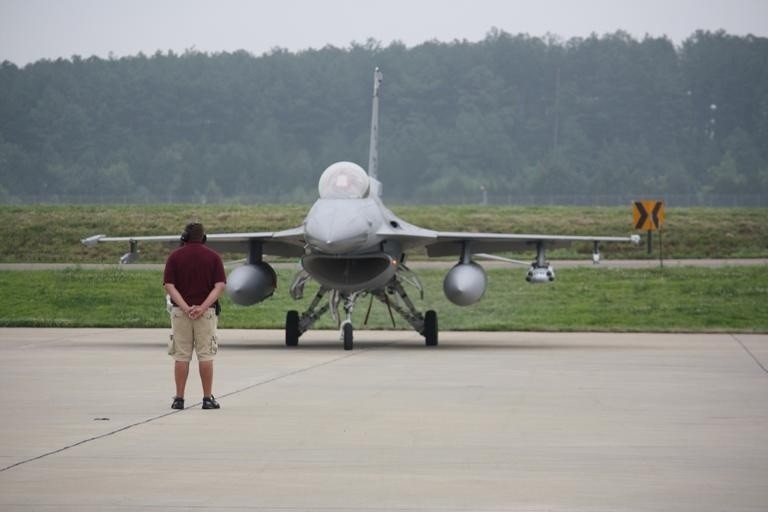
[81, 66, 641, 350]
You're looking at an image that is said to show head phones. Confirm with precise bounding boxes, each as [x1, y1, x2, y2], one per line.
[181, 222, 207, 244]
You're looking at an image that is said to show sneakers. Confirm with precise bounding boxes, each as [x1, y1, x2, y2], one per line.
[202, 394, 220, 409]
[171, 398, 184, 409]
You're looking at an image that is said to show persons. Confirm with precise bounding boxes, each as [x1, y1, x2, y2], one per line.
[162, 223, 225, 409]
[166, 295, 175, 356]
